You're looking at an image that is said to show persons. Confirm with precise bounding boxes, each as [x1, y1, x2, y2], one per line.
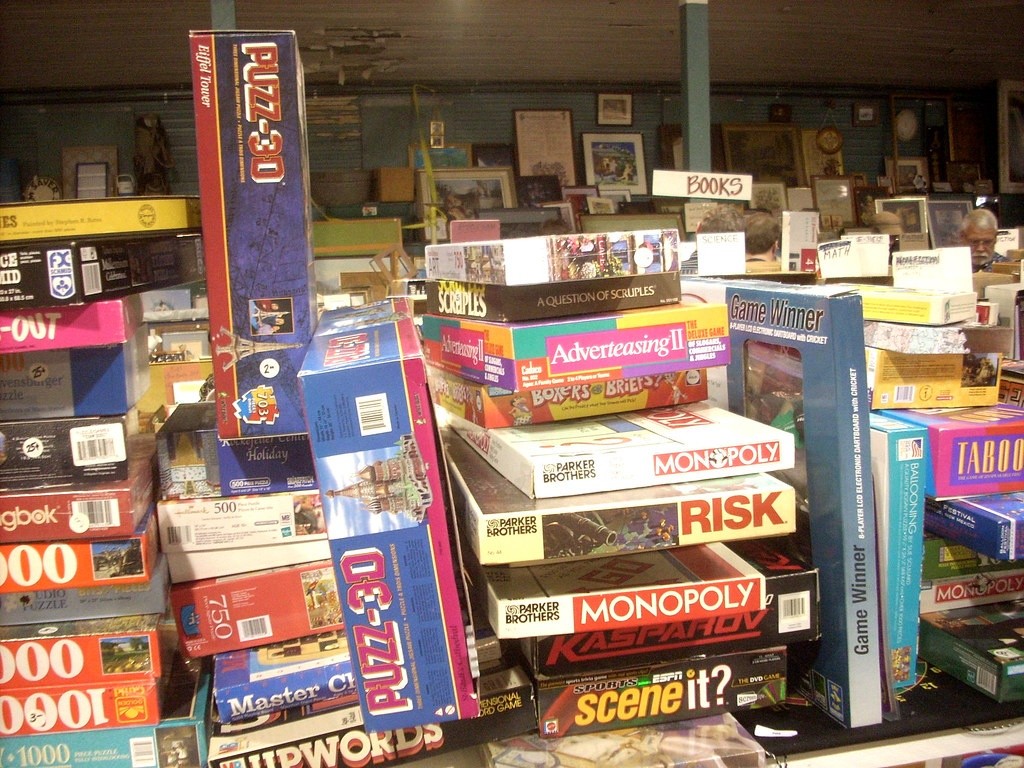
[958, 209, 1008, 274]
[870, 212, 903, 258]
[676, 208, 783, 274]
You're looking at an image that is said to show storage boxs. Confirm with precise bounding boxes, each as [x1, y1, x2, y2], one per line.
[0, 199, 1024, 767]
[373, 167, 415, 200]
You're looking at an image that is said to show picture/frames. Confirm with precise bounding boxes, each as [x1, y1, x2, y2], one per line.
[407, 80, 1024, 255]
[77, 162, 108, 198]
